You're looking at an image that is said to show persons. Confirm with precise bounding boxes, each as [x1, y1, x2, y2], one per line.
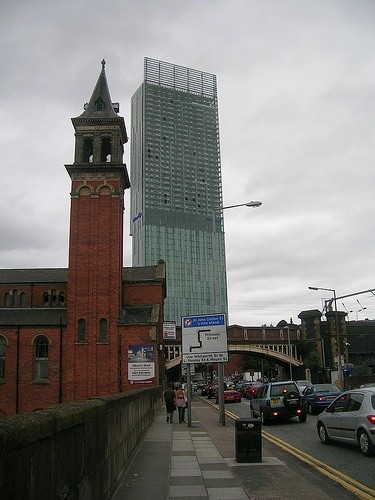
[164, 384, 177, 423]
[174, 387, 187, 423]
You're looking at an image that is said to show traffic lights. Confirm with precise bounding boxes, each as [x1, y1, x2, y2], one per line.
[213, 371, 216, 378]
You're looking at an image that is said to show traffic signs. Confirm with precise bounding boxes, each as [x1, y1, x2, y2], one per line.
[182, 313, 227, 363]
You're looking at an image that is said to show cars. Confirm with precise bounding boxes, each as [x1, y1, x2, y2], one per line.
[192, 379, 281, 403]
[315, 389, 375, 457]
[291, 380, 341, 415]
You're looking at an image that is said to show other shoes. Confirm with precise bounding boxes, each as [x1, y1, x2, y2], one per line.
[166, 417, 169, 423]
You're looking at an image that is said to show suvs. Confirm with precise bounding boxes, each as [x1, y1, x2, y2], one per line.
[250, 381, 306, 426]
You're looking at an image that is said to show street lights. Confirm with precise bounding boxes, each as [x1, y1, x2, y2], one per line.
[284, 326, 293, 387]
[212, 201, 263, 423]
[308, 287, 343, 392]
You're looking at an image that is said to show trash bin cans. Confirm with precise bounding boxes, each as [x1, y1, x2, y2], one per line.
[234, 418, 263, 464]
